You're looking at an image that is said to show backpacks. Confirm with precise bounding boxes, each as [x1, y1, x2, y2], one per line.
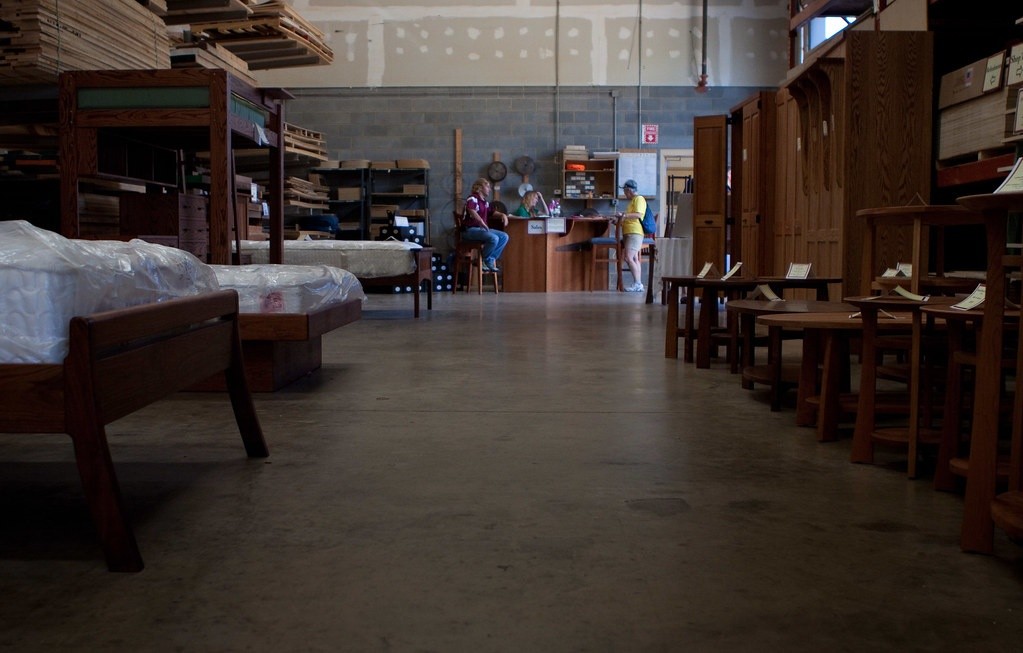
[633, 195, 656, 233]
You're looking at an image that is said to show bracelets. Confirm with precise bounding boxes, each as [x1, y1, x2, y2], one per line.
[622, 213, 624, 217]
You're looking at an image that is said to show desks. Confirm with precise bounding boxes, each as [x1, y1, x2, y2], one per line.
[856, 203, 976, 295]
[955, 191, 1023, 557]
[661, 275, 1023, 491]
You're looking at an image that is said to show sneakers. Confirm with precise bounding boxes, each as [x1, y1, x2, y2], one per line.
[624, 283, 644, 292]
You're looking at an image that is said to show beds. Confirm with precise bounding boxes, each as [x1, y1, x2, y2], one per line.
[0, 219, 270, 573]
[232, 239, 434, 319]
[180, 262, 370, 394]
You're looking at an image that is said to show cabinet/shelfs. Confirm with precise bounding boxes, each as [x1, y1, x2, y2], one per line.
[366, 167, 431, 245]
[689, 29, 932, 303]
[306, 167, 366, 240]
[561, 159, 617, 200]
[57, 67, 285, 265]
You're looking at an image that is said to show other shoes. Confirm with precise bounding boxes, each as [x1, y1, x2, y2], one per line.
[473, 260, 489, 271]
[483, 259, 499, 272]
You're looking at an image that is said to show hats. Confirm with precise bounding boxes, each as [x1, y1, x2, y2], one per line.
[619, 179, 637, 190]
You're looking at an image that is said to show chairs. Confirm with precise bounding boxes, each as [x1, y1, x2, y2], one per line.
[589, 216, 624, 292]
[452, 210, 499, 296]
[620, 214, 659, 291]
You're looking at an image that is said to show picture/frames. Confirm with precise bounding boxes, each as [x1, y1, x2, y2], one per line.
[1012, 88, 1023, 135]
[982, 49, 1007, 94]
[1005, 40, 1023, 87]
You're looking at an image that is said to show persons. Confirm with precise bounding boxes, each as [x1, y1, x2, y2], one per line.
[459, 177, 509, 273]
[609, 179, 646, 292]
[514, 190, 550, 217]
